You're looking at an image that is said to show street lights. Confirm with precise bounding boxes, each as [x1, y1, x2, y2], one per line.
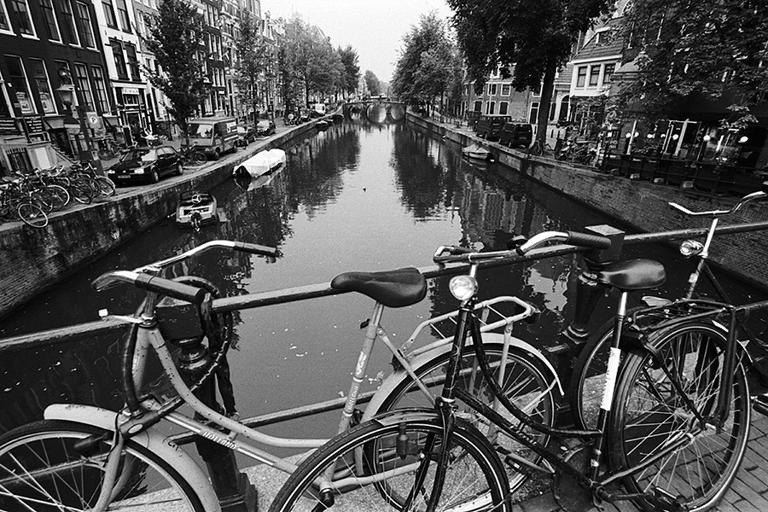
[445, 92, 448, 121]
[477, 95, 483, 115]
[224, 96, 231, 116]
[57, 84, 87, 163]
[589, 125, 749, 193]
[268, 96, 275, 123]
[563, 93, 576, 142]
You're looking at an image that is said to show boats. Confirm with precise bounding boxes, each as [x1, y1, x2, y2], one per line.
[459, 142, 495, 164]
[315, 112, 343, 133]
[231, 146, 287, 178]
[175, 189, 218, 233]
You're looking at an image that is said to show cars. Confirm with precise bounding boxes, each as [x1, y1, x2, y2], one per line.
[282, 103, 337, 126]
[256, 119, 275, 136]
[234, 123, 256, 147]
[106, 145, 183, 185]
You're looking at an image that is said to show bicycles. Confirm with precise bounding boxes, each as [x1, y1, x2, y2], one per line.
[0, 162, 116, 228]
[266, 229, 754, 512]
[571, 191, 768, 443]
[176, 139, 207, 166]
[0, 238, 567, 512]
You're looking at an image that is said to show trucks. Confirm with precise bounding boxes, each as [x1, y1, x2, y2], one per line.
[176, 115, 238, 158]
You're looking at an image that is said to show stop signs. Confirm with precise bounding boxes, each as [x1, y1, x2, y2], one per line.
[63, 116, 81, 135]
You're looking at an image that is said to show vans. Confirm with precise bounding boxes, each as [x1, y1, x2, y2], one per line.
[496, 121, 532, 149]
[474, 114, 511, 141]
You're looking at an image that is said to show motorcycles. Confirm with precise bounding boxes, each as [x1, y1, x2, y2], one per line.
[556, 136, 595, 167]
